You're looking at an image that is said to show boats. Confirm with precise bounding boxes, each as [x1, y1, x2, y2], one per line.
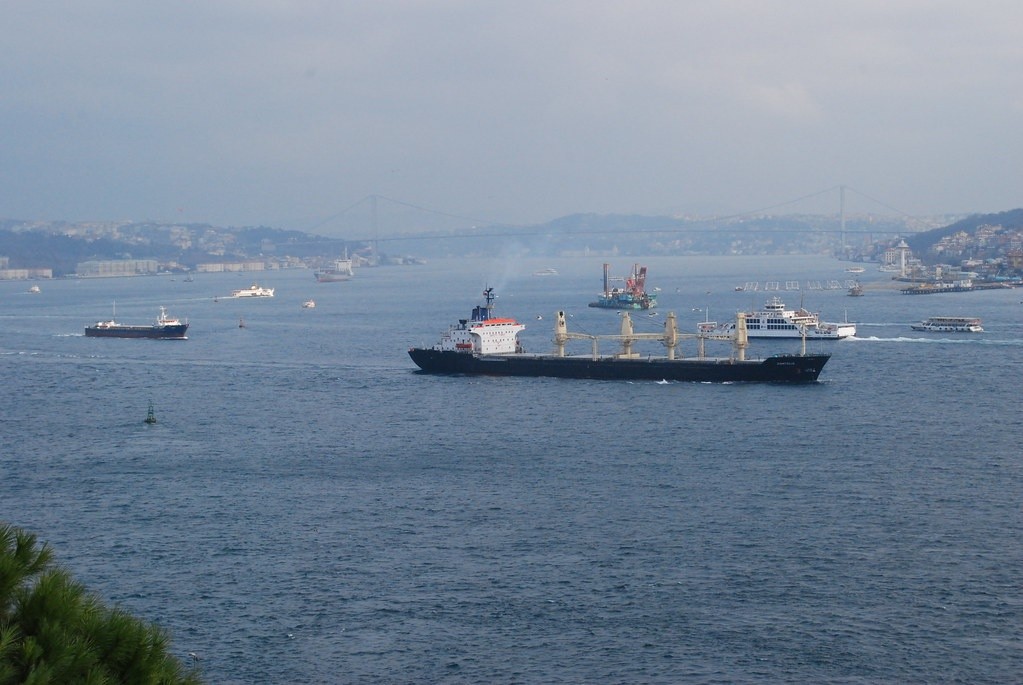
[712, 294, 857, 339]
[589, 263, 658, 309]
[455, 343, 472, 349]
[847, 280, 864, 297]
[697, 306, 718, 335]
[314, 258, 354, 283]
[407, 282, 831, 386]
[844, 266, 867, 273]
[85, 299, 190, 340]
[910, 317, 984, 331]
[232, 284, 275, 298]
